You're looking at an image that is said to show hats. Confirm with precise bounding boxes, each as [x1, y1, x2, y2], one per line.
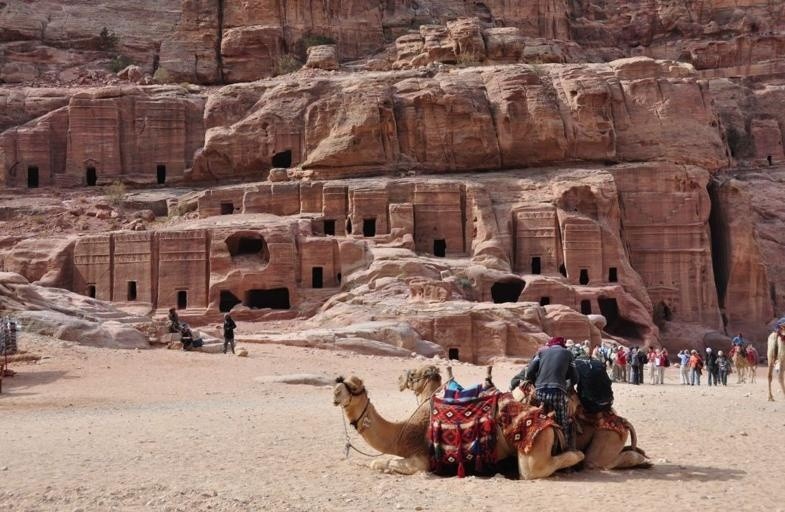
[548, 336, 565, 346]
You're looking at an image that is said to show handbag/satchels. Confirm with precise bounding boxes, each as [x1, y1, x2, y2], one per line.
[665, 357, 669, 367]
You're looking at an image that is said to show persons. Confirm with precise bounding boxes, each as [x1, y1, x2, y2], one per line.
[167, 307, 179, 333]
[523, 332, 747, 474]
[221, 312, 237, 355]
[180, 324, 193, 352]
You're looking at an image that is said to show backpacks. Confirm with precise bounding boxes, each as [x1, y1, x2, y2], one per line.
[641, 353, 646, 363]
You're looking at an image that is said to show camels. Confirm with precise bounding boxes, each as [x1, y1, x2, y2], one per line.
[330, 374, 585, 481]
[767, 322, 785, 402]
[731, 344, 759, 385]
[398, 364, 655, 470]
